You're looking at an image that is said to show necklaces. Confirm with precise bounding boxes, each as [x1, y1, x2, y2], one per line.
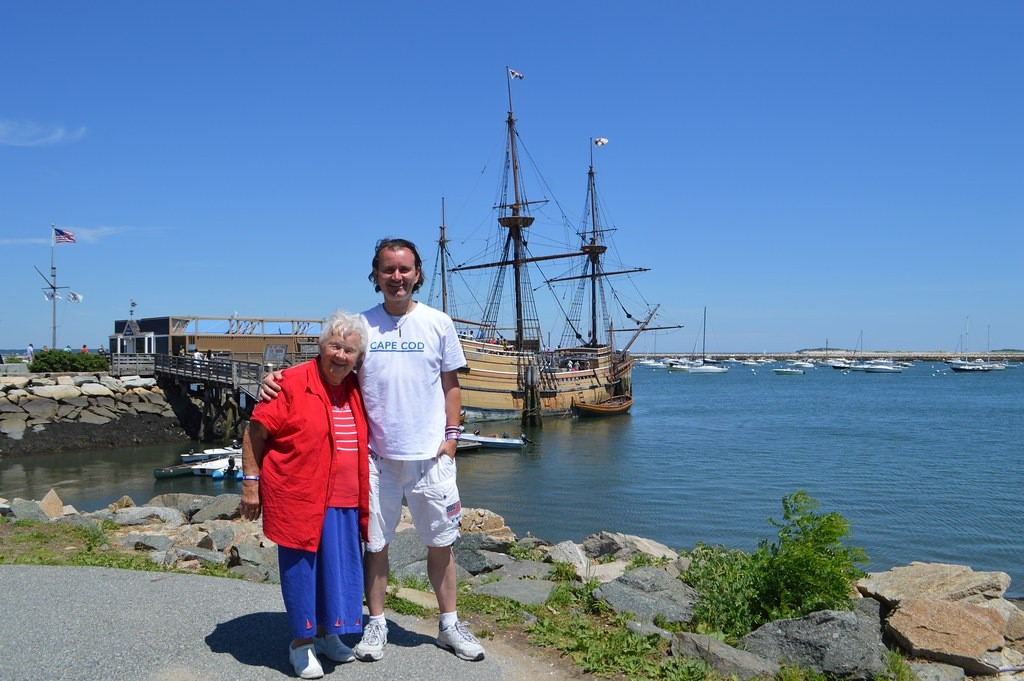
[383, 299, 416, 338]
[328, 382, 346, 411]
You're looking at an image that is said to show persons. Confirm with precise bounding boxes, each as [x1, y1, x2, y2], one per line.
[26, 343, 87, 364]
[502, 432, 510, 438]
[462, 330, 510, 356]
[192, 348, 204, 376]
[240, 308, 371, 678]
[474, 427, 481, 436]
[179, 345, 185, 370]
[257, 239, 485, 661]
[566, 360, 591, 371]
[203, 349, 216, 375]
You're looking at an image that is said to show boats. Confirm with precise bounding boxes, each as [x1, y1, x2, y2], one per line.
[454, 410, 535, 451]
[154, 439, 243, 480]
[633, 307, 1024, 376]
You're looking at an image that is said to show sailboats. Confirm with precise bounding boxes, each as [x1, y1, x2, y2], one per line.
[428, 63, 687, 443]
[569, 318, 635, 415]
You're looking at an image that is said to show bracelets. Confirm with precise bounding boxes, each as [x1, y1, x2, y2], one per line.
[243, 475, 259, 480]
[445, 425, 462, 434]
[445, 432, 462, 441]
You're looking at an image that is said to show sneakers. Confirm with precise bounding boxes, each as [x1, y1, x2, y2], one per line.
[356, 624, 386, 662]
[437, 622, 486, 662]
[290, 639, 324, 677]
[312, 635, 355, 663]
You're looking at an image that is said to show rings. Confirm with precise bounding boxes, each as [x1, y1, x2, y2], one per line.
[240, 505, 244, 508]
[245, 506, 247, 510]
[249, 510, 255, 512]
[256, 508, 258, 512]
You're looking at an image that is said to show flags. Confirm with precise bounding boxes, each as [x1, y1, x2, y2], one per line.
[55, 228, 76, 243]
